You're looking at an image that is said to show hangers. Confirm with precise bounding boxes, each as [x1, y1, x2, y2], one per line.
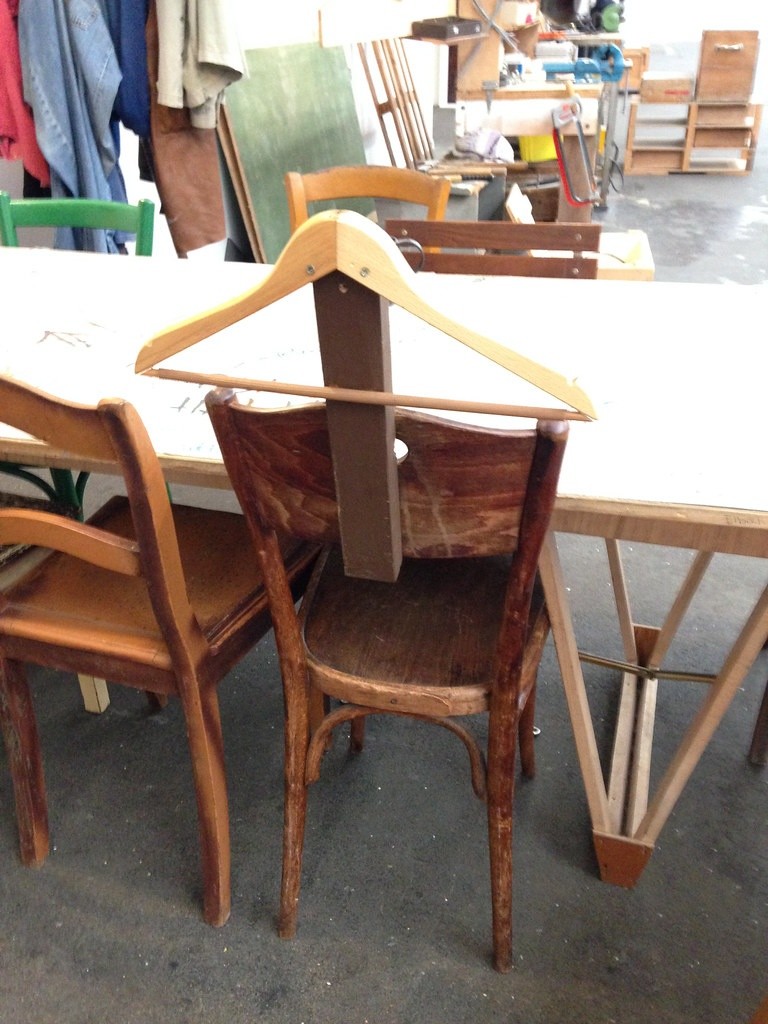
[132, 207, 597, 423]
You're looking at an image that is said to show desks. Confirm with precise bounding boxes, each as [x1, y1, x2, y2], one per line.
[0, 247, 768, 887]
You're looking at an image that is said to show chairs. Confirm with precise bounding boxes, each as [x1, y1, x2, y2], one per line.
[387, 216, 602, 278]
[0, 374, 332, 929]
[0, 190, 173, 525]
[505, 184, 655, 283]
[202, 387, 567, 971]
[284, 164, 452, 272]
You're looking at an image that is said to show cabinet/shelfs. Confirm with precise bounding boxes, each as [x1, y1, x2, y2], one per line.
[455, 1, 763, 224]
